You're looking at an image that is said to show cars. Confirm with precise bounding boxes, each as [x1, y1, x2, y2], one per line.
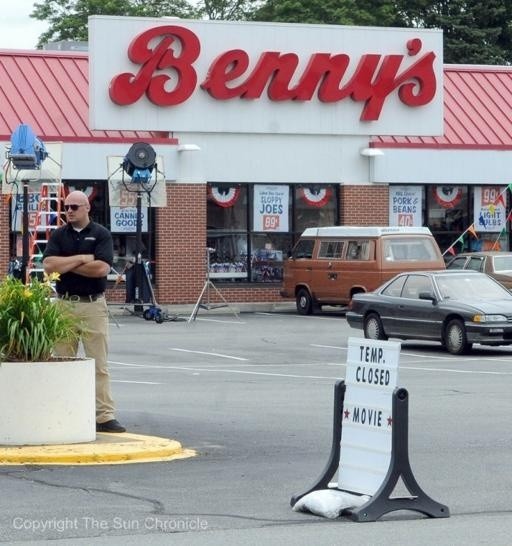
[346, 251, 512, 355]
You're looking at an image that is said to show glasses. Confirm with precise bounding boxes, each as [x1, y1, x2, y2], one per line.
[64, 205, 86, 211]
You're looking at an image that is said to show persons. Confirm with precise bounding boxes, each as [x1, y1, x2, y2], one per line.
[42, 190, 126, 432]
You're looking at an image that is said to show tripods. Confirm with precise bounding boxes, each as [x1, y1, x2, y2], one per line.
[186, 254, 241, 322]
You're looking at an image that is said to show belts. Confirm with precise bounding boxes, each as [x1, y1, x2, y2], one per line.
[58, 293, 106, 303]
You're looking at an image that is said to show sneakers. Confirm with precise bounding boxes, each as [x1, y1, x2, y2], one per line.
[96, 419, 126, 433]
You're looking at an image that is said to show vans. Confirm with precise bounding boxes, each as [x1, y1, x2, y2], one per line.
[280, 227, 446, 315]
